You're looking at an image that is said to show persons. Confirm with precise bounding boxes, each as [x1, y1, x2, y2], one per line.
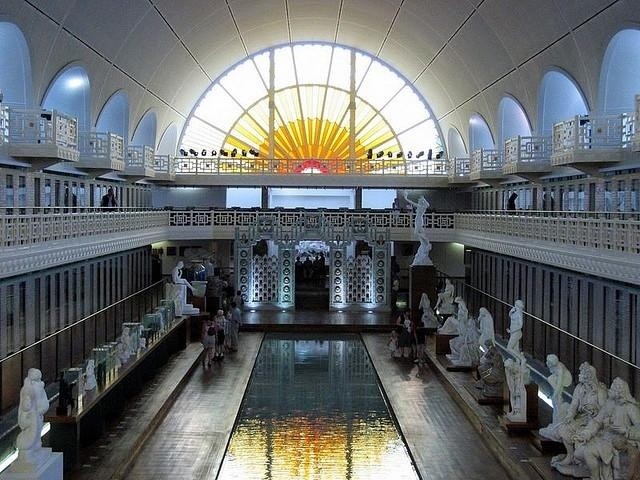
[402, 187, 431, 245]
[541, 193, 555, 212]
[171, 260, 198, 294]
[505, 193, 518, 214]
[82, 359, 98, 389]
[100, 188, 120, 213]
[192, 256, 245, 369]
[503, 299, 526, 421]
[120, 327, 132, 359]
[295, 252, 326, 288]
[543, 353, 637, 480]
[389, 308, 414, 357]
[420, 278, 505, 384]
